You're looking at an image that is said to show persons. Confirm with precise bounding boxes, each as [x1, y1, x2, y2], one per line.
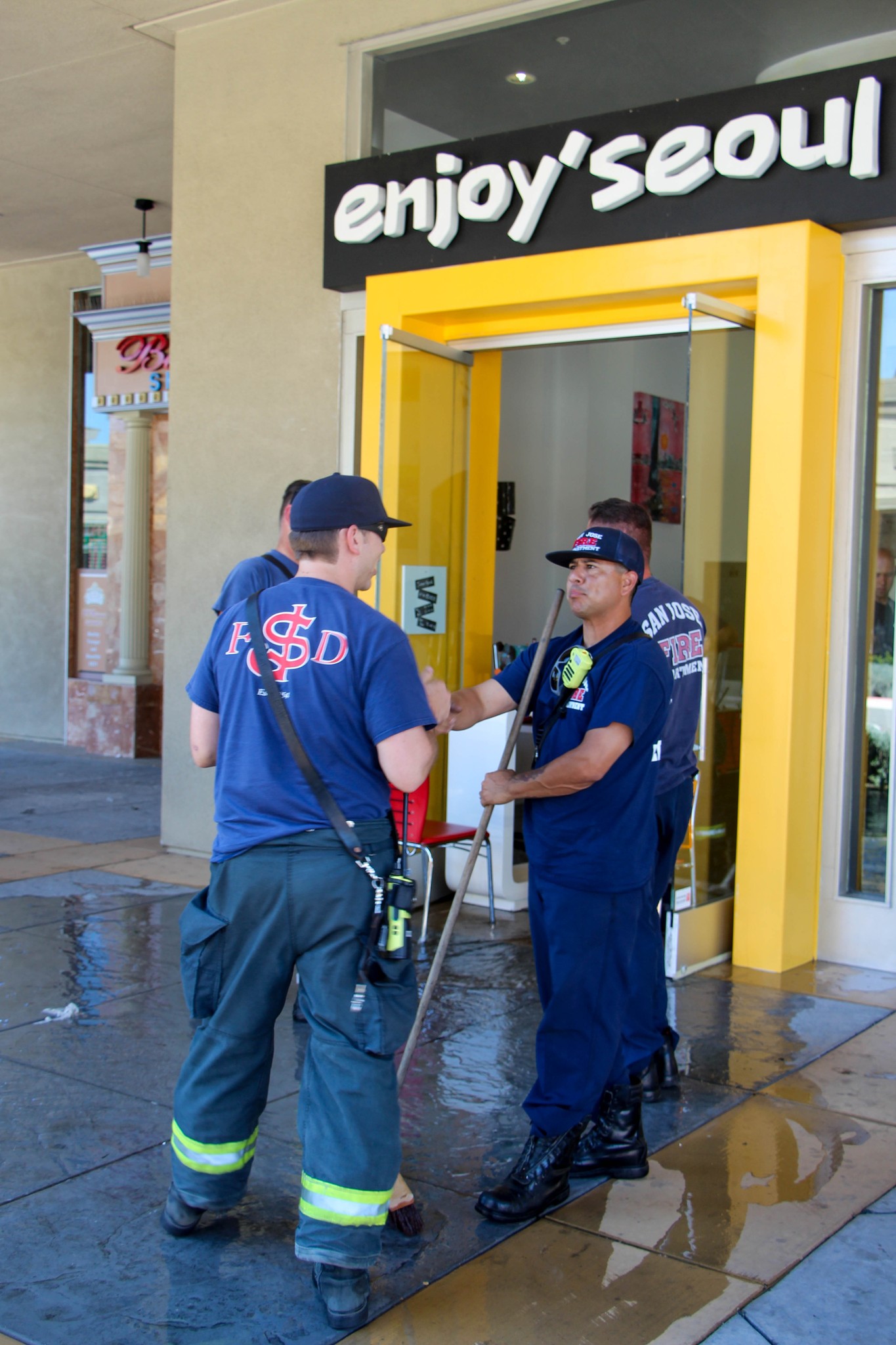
[162, 473, 450, 1327]
[212, 480, 311, 616]
[586, 498, 706, 1103]
[440, 527, 672, 1224]
[874, 548, 896, 658]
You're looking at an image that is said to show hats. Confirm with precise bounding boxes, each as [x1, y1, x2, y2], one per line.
[545, 524, 645, 582]
[289, 471, 417, 533]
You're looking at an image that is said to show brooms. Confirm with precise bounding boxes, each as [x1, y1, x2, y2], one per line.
[387, 587, 565, 1238]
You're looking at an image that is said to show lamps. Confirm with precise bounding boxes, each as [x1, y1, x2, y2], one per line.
[131, 197, 158, 278]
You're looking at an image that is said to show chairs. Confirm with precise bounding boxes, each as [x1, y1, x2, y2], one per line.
[390, 770, 496, 946]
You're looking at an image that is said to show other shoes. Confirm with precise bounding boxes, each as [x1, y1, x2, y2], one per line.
[307, 1257, 371, 1329]
[162, 1178, 209, 1238]
[633, 1044, 682, 1102]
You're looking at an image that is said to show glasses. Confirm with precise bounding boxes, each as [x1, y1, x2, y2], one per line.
[359, 524, 390, 541]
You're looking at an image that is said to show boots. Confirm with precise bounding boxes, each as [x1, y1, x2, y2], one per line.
[570, 1078, 650, 1189]
[471, 1116, 594, 1221]
